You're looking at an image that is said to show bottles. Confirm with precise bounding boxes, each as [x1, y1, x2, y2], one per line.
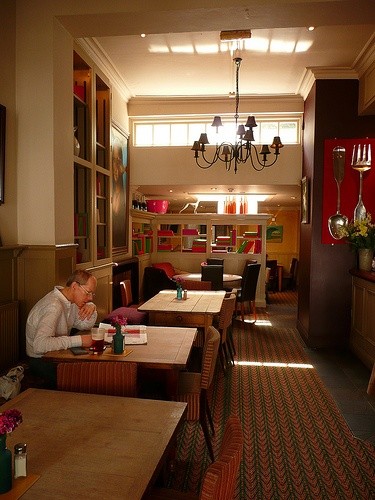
[223, 195, 249, 214]
[132, 194, 147, 212]
[12, 443, 28, 479]
[183, 289, 187, 300]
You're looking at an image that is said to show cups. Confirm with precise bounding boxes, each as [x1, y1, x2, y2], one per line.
[90, 327, 105, 355]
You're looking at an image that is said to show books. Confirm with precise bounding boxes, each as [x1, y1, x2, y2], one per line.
[99, 323, 147, 345]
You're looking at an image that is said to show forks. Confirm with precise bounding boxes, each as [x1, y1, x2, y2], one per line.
[349, 142, 372, 228]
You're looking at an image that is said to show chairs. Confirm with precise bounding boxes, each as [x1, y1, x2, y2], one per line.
[55, 257, 299, 500]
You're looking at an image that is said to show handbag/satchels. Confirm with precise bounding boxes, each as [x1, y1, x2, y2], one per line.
[0, 366, 24, 400]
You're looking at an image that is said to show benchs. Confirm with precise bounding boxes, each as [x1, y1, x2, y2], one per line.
[113, 261, 149, 325]
[0, 300, 53, 407]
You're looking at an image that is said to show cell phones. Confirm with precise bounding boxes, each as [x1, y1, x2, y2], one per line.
[70, 348, 90, 355]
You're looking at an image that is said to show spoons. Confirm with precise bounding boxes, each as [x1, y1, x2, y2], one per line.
[328, 145, 349, 240]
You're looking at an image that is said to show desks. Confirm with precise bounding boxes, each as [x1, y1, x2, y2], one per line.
[138, 289, 227, 338]
[173, 272, 242, 286]
[0, 388, 189, 500]
[41, 322, 199, 370]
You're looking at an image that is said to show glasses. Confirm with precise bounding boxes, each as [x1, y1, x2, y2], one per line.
[75, 280, 96, 296]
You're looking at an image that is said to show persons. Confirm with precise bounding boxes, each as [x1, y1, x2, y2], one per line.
[25, 270, 98, 386]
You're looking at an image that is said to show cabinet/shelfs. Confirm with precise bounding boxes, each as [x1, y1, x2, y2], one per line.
[72, 49, 112, 270]
[131, 209, 267, 307]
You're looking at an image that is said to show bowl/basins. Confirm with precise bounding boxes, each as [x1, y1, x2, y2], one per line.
[146, 200, 169, 214]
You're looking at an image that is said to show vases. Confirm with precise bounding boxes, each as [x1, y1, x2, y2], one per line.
[0, 433, 14, 494]
[359, 249, 373, 271]
[177, 284, 182, 299]
[113, 325, 125, 354]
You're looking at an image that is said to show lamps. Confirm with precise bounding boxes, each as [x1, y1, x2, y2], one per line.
[189, 58, 286, 175]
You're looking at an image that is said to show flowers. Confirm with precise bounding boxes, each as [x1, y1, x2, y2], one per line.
[201, 261, 207, 266]
[176, 276, 184, 285]
[110, 315, 128, 328]
[334, 212, 375, 254]
[0, 408, 23, 434]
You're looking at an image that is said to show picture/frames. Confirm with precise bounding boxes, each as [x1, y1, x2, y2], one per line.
[111, 122, 130, 258]
[301, 176, 310, 224]
